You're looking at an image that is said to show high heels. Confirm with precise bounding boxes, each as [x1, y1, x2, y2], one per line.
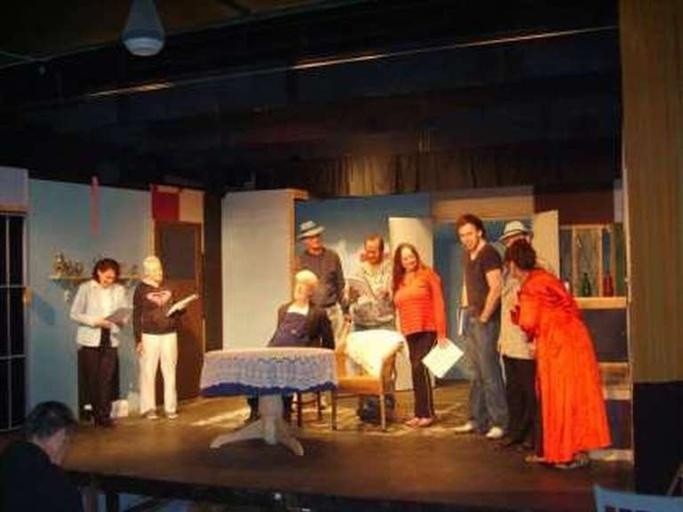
[141, 412, 161, 421]
[165, 410, 179, 420]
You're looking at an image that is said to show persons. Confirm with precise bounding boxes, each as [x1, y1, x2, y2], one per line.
[243, 270, 332, 417]
[390, 243, 448, 428]
[133, 255, 189, 421]
[448, 213, 513, 442]
[68, 258, 133, 428]
[0, 402, 84, 511]
[493, 219, 538, 454]
[292, 221, 348, 352]
[348, 233, 396, 425]
[503, 238, 615, 471]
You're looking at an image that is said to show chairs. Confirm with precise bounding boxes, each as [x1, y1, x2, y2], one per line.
[288, 300, 344, 427]
[329, 329, 403, 431]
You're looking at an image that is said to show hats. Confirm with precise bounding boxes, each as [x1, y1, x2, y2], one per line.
[294, 219, 327, 241]
[498, 219, 534, 242]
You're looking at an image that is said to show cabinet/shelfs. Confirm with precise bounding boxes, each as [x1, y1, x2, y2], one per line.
[560, 224, 631, 403]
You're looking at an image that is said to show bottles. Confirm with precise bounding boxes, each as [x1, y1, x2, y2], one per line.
[580, 273, 590, 297]
[604, 271, 614, 297]
[564, 278, 571, 294]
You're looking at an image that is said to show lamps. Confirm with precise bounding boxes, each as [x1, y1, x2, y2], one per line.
[121, 0, 166, 57]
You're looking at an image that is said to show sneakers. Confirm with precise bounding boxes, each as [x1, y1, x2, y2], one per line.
[95, 417, 117, 428]
[405, 413, 438, 427]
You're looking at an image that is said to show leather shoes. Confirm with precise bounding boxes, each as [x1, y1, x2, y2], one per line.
[552, 447, 591, 470]
[523, 451, 546, 463]
[451, 418, 481, 434]
[499, 432, 516, 447]
[485, 424, 505, 439]
[514, 438, 539, 452]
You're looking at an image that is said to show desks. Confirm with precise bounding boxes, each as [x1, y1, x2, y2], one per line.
[198, 348, 332, 456]
